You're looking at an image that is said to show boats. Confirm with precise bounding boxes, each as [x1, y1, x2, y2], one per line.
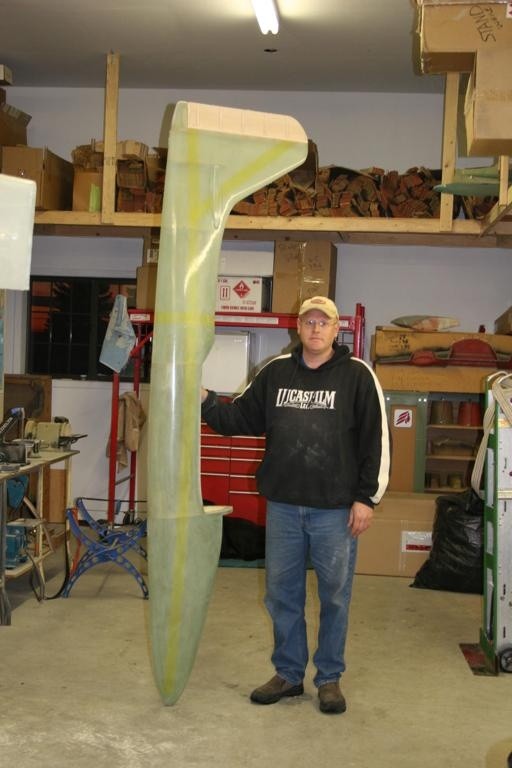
[145, 99, 309, 710]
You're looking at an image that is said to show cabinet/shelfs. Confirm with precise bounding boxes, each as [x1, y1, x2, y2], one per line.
[107, 307, 367, 533]
[418, 394, 487, 493]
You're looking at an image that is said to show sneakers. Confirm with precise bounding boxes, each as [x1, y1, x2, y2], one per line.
[318, 681, 347, 714]
[250, 673, 305, 706]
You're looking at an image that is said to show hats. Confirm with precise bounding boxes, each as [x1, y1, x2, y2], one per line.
[298, 295, 340, 321]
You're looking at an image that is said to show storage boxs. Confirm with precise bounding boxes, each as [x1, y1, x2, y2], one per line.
[135, 233, 160, 311]
[1, 102, 103, 213]
[352, 406, 442, 585]
[213, 237, 337, 317]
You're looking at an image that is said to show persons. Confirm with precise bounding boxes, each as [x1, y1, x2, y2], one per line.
[198, 296, 391, 713]
